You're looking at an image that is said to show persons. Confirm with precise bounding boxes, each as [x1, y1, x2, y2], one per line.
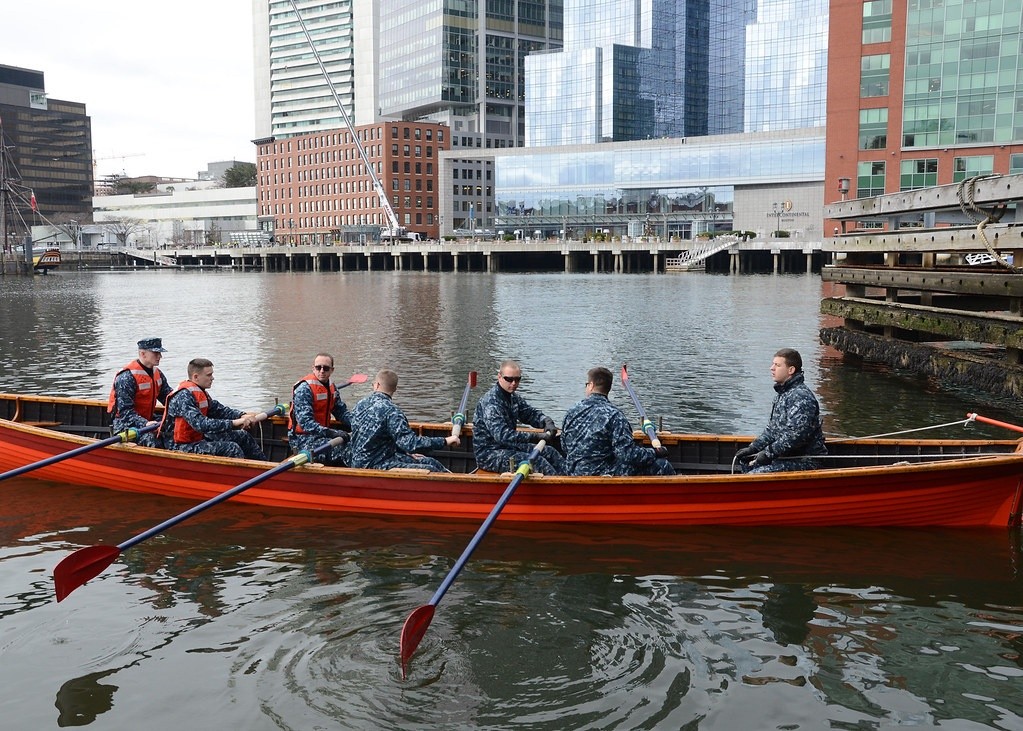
[343, 369, 461, 473]
[157, 359, 269, 461]
[734, 348, 827, 474]
[287, 352, 351, 468]
[107, 337, 173, 448]
[560, 367, 675, 476]
[471, 360, 565, 476]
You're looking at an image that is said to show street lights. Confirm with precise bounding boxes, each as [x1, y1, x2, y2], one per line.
[70, 220, 79, 251]
[591, 213, 595, 237]
[288, 220, 295, 243]
[772, 202, 785, 238]
[434, 215, 439, 240]
[708, 207, 720, 238]
[356, 218, 365, 246]
[647, 212, 650, 243]
[521, 213, 530, 240]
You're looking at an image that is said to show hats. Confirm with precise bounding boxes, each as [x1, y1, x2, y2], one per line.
[137, 338, 168, 352]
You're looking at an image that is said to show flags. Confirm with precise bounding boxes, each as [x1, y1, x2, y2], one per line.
[30, 189, 39, 214]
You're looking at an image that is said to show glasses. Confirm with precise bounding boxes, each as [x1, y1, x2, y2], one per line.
[585, 383, 590, 387]
[314, 365, 332, 371]
[501, 374, 521, 382]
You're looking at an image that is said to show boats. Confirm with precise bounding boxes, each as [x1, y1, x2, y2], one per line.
[1, 389, 1022, 534]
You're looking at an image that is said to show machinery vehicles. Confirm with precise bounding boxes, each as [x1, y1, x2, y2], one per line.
[291, 0, 420, 243]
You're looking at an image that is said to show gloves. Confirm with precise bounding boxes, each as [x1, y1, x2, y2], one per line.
[326, 429, 351, 446]
[652, 446, 668, 461]
[735, 447, 758, 464]
[543, 417, 558, 438]
[529, 432, 552, 445]
[748, 448, 772, 469]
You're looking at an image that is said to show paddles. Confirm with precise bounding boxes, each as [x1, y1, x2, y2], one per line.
[240, 373, 368, 430]
[53, 430, 353, 604]
[450, 371, 478, 447]
[620, 364, 668, 459]
[0, 421, 165, 485]
[400, 430, 552, 683]
